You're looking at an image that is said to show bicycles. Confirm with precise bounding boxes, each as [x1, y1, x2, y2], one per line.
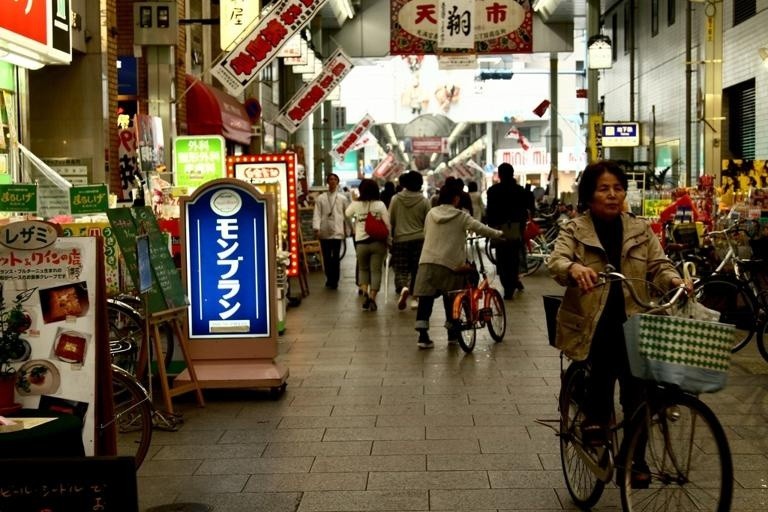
[108, 292, 174, 473]
[340, 219, 349, 260]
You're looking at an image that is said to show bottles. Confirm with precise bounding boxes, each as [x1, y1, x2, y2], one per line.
[644, 188, 672, 215]
[731, 189, 748, 204]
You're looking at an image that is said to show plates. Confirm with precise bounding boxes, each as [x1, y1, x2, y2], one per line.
[17, 359, 60, 397]
[17, 306, 37, 336]
[53, 331, 88, 363]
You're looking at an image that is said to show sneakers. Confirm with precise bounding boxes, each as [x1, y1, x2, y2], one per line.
[580, 417, 608, 447]
[447, 328, 459, 344]
[630, 461, 651, 490]
[358, 289, 378, 311]
[396, 287, 419, 311]
[416, 333, 435, 349]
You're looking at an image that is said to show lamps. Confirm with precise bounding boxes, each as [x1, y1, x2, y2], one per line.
[586, 0, 625, 68]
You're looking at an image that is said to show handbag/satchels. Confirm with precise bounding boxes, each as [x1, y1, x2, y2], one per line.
[524, 208, 541, 240]
[365, 200, 389, 242]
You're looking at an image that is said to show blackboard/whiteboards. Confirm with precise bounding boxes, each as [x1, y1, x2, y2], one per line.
[106, 206, 190, 314]
[0, 456, 140, 512]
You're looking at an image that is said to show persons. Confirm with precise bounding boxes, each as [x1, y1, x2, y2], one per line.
[480, 162, 532, 301]
[344, 172, 535, 311]
[543, 160, 698, 489]
[410, 183, 507, 350]
[311, 173, 354, 289]
